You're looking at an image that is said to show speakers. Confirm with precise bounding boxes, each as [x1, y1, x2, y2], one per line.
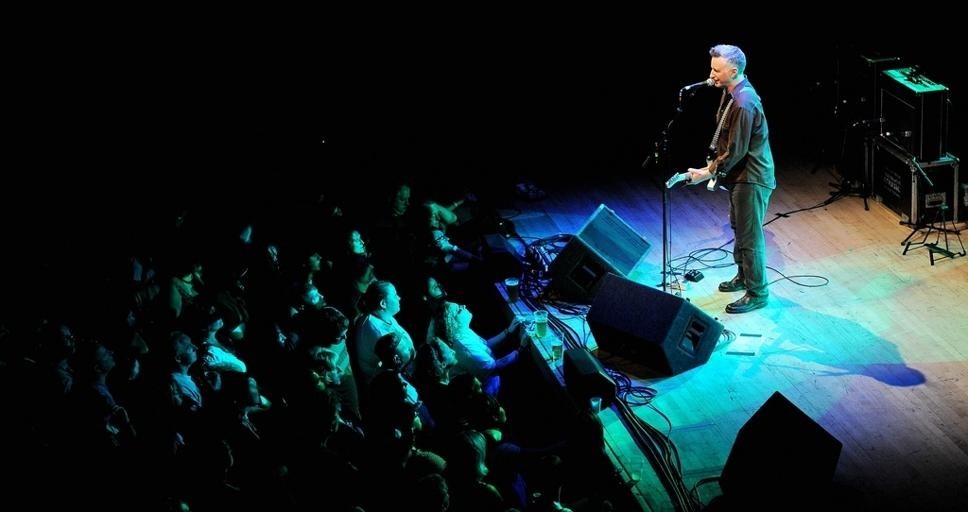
[548, 204, 654, 305]
[586, 272, 724, 377]
[880, 67, 951, 163]
[846, 52, 904, 140]
[719, 391, 843, 512]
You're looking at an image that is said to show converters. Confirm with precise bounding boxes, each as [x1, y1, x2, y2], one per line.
[685, 269, 704, 282]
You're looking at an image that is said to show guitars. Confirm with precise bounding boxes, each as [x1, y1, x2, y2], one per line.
[665, 148, 728, 192]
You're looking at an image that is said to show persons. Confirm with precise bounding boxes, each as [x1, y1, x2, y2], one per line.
[38, 182, 622, 512]
[685, 43, 776, 314]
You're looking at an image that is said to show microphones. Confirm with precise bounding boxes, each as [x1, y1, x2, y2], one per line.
[682, 78, 715, 92]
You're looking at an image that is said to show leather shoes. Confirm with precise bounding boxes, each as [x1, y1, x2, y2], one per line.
[725, 292, 769, 314]
[718, 274, 746, 292]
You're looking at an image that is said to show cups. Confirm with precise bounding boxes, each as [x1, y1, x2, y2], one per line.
[505, 277, 520, 303]
[590, 397, 602, 415]
[550, 331, 564, 361]
[534, 310, 548, 339]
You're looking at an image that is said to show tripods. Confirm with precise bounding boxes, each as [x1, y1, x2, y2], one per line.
[824, 144, 869, 211]
[881, 133, 961, 246]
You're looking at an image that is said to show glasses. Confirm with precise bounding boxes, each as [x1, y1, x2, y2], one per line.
[436, 234, 445, 241]
[455, 304, 466, 319]
